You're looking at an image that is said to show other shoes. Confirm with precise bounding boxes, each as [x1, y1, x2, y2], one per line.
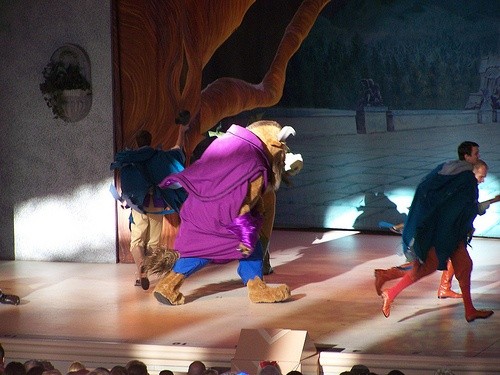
[465, 311, 493, 323]
[380, 289, 392, 318]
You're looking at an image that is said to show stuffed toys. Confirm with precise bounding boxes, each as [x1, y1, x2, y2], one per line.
[153, 119, 303, 306]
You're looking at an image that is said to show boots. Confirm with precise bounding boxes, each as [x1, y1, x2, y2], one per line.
[375, 267, 403, 296]
[131, 246, 150, 291]
[437, 258, 464, 299]
[134, 250, 155, 287]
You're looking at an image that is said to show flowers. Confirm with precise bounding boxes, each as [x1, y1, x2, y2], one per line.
[39, 54, 92, 120]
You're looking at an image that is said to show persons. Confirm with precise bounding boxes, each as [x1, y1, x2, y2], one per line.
[0, 344, 407, 375]
[380, 141, 500, 323]
[375, 159, 487, 299]
[110, 111, 192, 293]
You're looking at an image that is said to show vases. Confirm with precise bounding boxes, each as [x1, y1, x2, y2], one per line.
[41, 90, 93, 121]
